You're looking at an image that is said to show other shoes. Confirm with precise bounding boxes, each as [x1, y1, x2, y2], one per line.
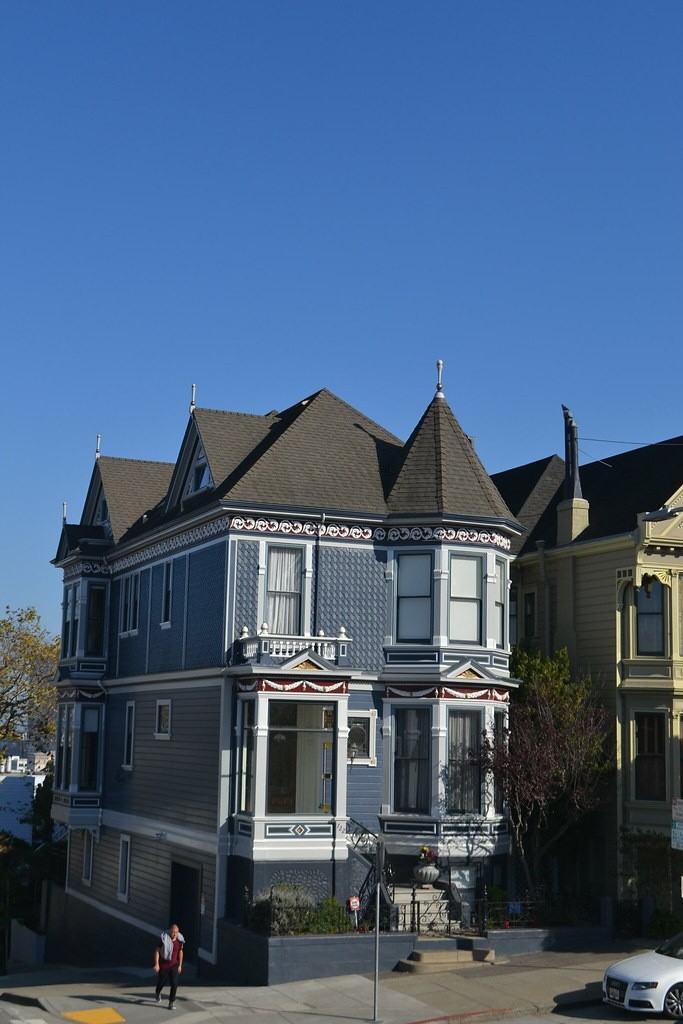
[156, 996, 161, 1001]
[168, 1003, 177, 1010]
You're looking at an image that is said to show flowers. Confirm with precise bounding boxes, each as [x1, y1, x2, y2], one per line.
[416, 846, 437, 868]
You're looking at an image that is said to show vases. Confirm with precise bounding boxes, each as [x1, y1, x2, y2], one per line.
[413, 862, 439, 883]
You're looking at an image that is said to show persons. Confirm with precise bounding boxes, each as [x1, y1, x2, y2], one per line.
[153, 924, 184, 1009]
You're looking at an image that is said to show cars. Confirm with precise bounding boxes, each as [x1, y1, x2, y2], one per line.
[601, 929, 683, 1022]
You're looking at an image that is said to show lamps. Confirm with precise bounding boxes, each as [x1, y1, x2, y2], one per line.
[348, 742, 358, 774]
